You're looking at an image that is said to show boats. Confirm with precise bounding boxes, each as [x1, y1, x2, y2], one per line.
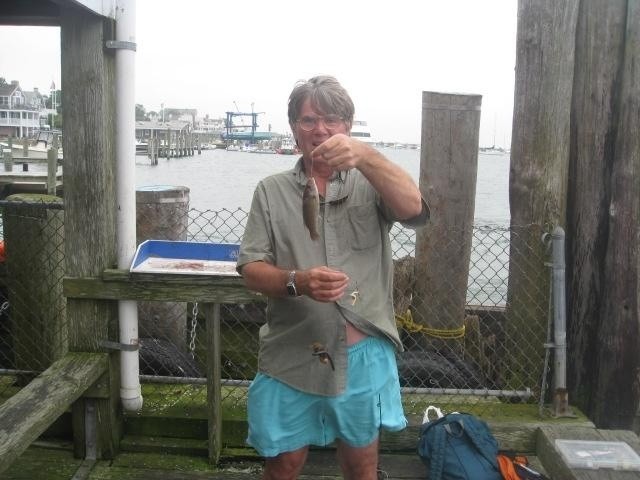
[0, 126, 64, 165]
[136, 135, 304, 157]
[381, 141, 507, 155]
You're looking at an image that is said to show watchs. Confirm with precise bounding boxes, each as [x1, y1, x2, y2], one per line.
[287, 271, 303, 298]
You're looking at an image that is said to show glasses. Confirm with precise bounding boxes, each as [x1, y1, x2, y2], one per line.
[293, 113, 346, 132]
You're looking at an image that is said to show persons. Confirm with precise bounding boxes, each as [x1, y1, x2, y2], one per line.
[235, 75, 422, 480]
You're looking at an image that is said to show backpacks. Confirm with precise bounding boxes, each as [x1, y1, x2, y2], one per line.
[418, 410, 529, 480]
